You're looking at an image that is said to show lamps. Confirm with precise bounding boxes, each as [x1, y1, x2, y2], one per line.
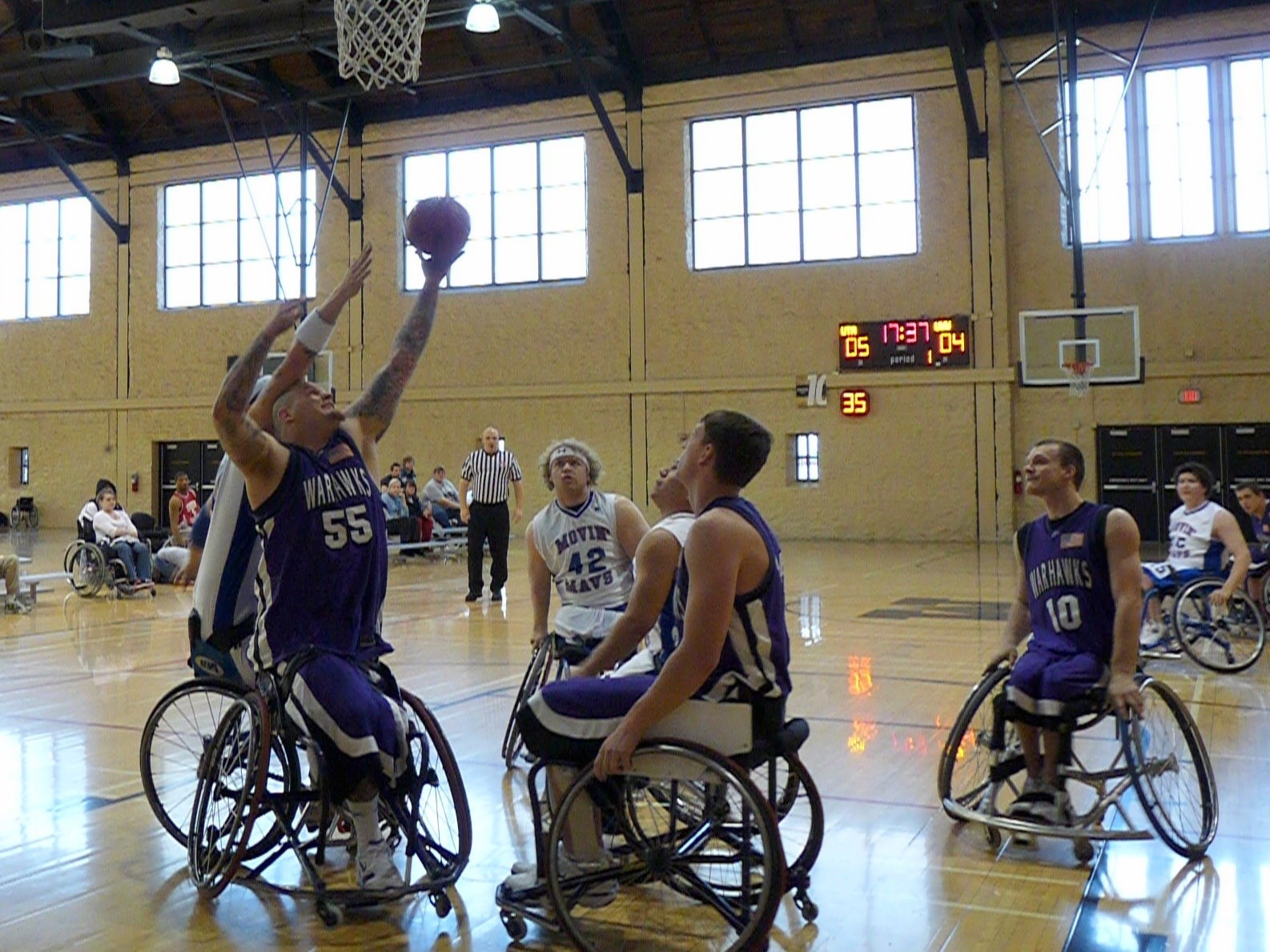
[465, 0, 500, 33]
[148, 45, 180, 85]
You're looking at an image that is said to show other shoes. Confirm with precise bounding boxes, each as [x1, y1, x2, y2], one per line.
[491, 589, 501, 601]
[464, 589, 482, 602]
[131, 578, 153, 585]
[402, 554, 425, 564]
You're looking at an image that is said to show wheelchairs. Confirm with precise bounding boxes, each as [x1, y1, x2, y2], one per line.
[64, 538, 156, 600]
[1137, 544, 1270, 672]
[9, 496, 38, 528]
[140, 608, 472, 927]
[938, 662, 1218, 862]
[495, 631, 824, 952]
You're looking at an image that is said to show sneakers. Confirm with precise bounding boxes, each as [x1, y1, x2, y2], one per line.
[355, 842, 404, 891]
[301, 801, 338, 832]
[1140, 622, 1167, 650]
[1009, 778, 1046, 814]
[3, 600, 33, 616]
[502, 855, 618, 907]
[1030, 780, 1073, 828]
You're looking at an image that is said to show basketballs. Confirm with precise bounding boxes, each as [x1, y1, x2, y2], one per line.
[406, 196, 472, 256]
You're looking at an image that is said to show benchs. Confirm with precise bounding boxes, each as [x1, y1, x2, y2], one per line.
[1, 557, 74, 601]
[386, 519, 493, 563]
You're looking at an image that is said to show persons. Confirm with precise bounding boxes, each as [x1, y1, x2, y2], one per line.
[1139, 464, 1251, 651]
[1226, 481, 1270, 631]
[75, 478, 155, 590]
[0, 553, 32, 614]
[459, 427, 524, 603]
[981, 439, 1147, 826]
[168, 236, 466, 902]
[501, 408, 789, 908]
